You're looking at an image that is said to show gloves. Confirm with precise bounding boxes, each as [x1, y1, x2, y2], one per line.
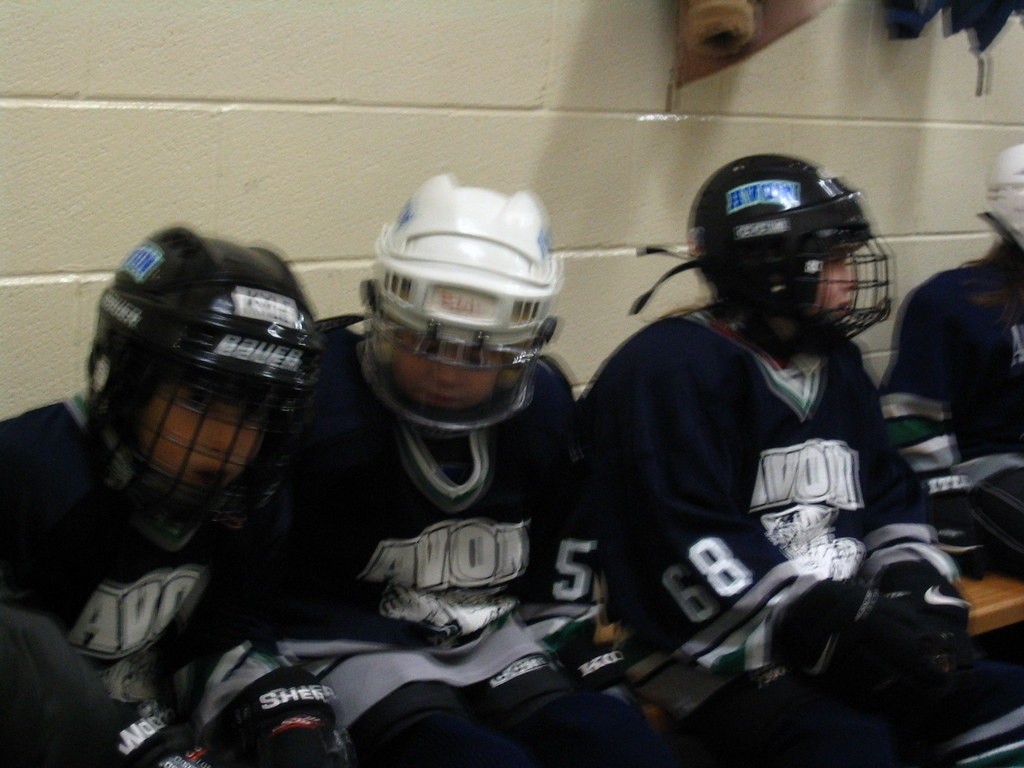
[791, 559, 970, 712]
[568, 647, 636, 707]
[220, 665, 341, 768]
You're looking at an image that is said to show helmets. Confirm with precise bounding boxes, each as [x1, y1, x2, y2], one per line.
[84, 228, 321, 525]
[372, 174, 560, 351]
[691, 155, 890, 339]
[985, 144, 1024, 249]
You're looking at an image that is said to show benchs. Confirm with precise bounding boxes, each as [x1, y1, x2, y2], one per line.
[593, 570, 1024, 731]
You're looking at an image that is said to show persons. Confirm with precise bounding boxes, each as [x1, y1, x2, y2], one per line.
[0, 227, 357, 767]
[877, 140, 1024, 580]
[276, 171, 654, 767]
[575, 151, 977, 768]
